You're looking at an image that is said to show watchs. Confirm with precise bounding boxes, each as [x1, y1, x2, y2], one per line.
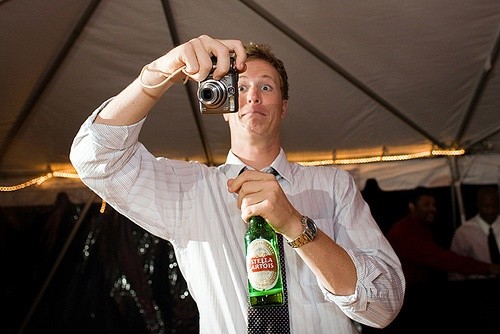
[286, 214, 319, 248]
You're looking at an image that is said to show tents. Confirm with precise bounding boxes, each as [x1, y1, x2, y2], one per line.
[0, 0, 500, 224]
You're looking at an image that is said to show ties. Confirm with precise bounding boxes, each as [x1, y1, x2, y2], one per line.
[239, 168, 290, 334]
[487, 227, 500, 277]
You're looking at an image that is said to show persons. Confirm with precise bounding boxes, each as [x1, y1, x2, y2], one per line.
[0, 191, 101, 289]
[449, 187, 500, 281]
[386, 187, 500, 334]
[69, 34, 406, 334]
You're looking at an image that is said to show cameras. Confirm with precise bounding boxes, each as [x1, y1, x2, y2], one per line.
[197, 57, 239, 114]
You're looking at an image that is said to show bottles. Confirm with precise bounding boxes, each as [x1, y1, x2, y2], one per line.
[245, 215, 284, 306]
